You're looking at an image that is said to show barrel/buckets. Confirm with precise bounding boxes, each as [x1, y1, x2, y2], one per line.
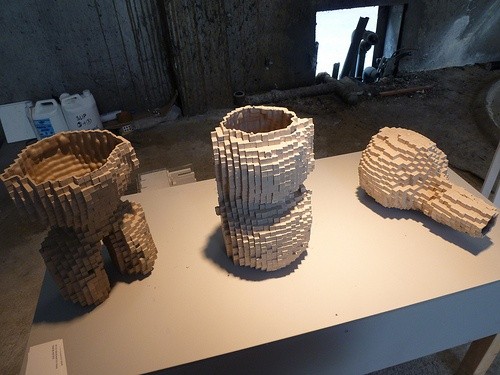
[61, 89, 103, 131]
[25, 99, 69, 141]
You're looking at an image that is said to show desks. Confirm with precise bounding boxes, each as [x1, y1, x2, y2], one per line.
[21, 148, 500, 375]
